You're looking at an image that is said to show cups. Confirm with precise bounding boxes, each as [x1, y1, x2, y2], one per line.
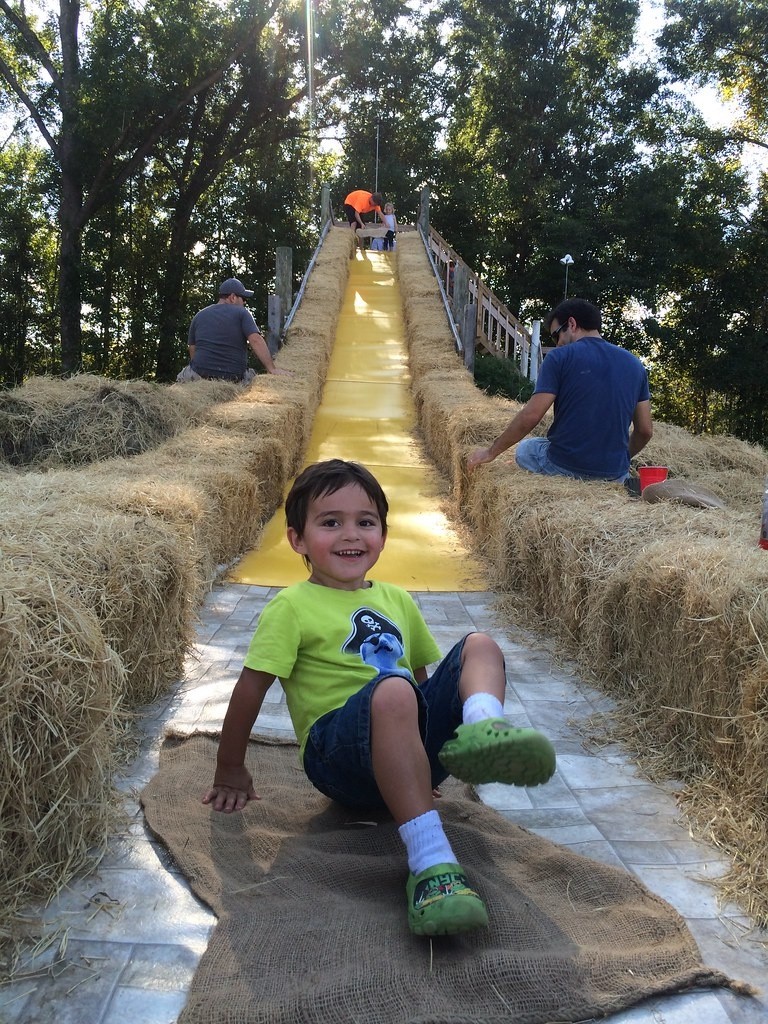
[639, 465, 669, 493]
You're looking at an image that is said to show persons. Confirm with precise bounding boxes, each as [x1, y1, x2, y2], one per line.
[343, 189, 390, 248]
[204, 461, 557, 942]
[371, 236, 397, 251]
[380, 201, 397, 252]
[177, 277, 284, 390]
[466, 296, 654, 482]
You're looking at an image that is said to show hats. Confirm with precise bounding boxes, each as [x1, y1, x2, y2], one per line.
[220, 278, 255, 297]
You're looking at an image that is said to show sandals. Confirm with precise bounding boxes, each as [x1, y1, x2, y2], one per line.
[405, 862, 490, 936]
[438, 716, 557, 787]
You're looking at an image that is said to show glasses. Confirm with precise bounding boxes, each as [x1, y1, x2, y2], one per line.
[547, 320, 568, 344]
[235, 294, 247, 302]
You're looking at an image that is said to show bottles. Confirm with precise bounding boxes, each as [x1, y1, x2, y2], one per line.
[759, 472, 768, 551]
